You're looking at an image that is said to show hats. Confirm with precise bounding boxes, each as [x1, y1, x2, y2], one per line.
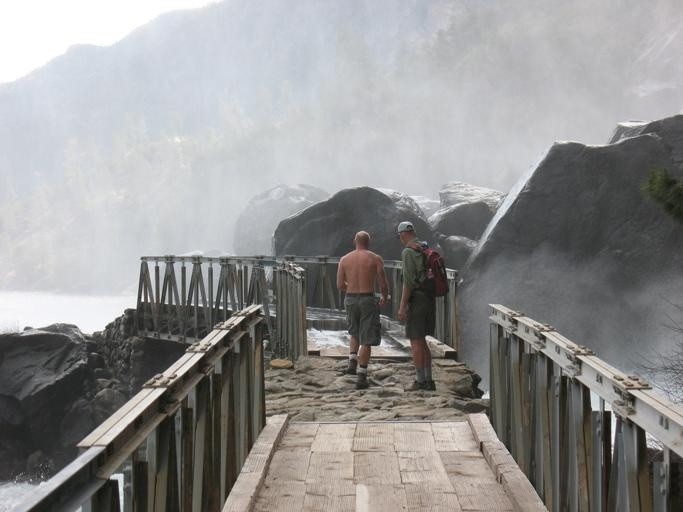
[397, 221, 415, 233]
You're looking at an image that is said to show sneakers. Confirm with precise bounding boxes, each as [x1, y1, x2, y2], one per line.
[355, 373, 369, 388]
[426, 380, 437, 391]
[402, 378, 427, 391]
[346, 360, 357, 375]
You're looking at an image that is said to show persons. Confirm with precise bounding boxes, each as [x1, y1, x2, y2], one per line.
[336, 231, 391, 390]
[395, 221, 437, 391]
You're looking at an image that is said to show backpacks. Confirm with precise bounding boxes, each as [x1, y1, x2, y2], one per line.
[404, 242, 449, 297]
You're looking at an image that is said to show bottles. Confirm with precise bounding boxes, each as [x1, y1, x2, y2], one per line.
[427, 268, 433, 279]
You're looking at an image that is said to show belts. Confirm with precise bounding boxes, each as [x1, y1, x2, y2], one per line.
[346, 292, 375, 297]
